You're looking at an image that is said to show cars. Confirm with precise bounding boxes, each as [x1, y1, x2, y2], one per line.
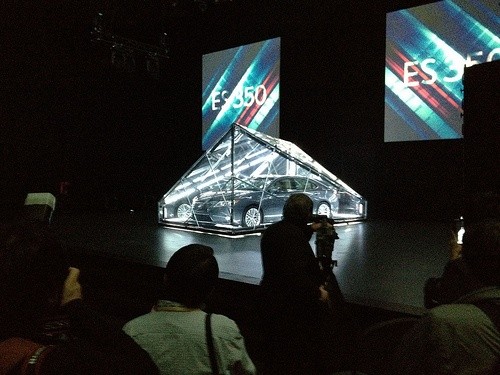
[192, 175, 339, 228]
[166, 179, 233, 219]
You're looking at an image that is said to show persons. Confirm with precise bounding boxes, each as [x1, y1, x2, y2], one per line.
[0, 192, 500, 375]
